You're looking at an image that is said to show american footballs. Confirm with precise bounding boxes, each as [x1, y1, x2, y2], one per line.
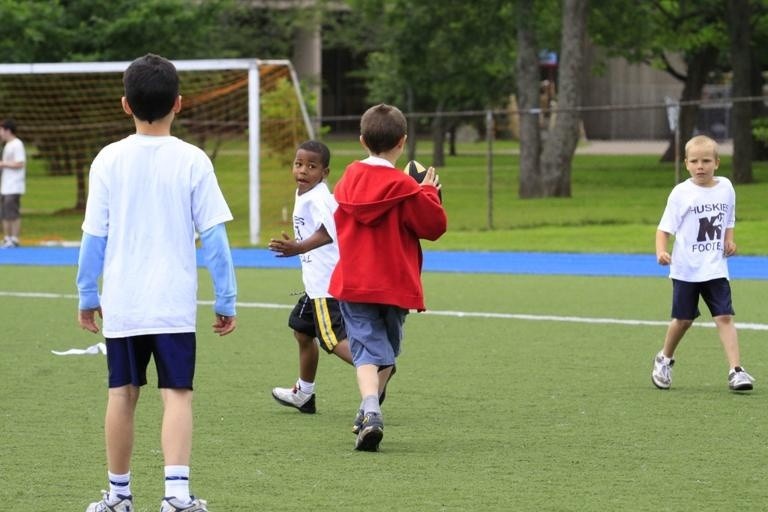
[402, 159, 442, 205]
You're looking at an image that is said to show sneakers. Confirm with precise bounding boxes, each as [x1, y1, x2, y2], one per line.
[272, 387, 315, 413]
[651, 352, 675, 389]
[728, 366, 755, 390]
[160, 495, 207, 512]
[87, 490, 132, 512]
[352, 409, 383, 449]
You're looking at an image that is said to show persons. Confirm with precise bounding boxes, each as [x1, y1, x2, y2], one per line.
[74, 51, 239, 510]
[324, 102, 448, 451]
[649, 135, 756, 390]
[266, 140, 398, 413]
[0, 120, 27, 249]
